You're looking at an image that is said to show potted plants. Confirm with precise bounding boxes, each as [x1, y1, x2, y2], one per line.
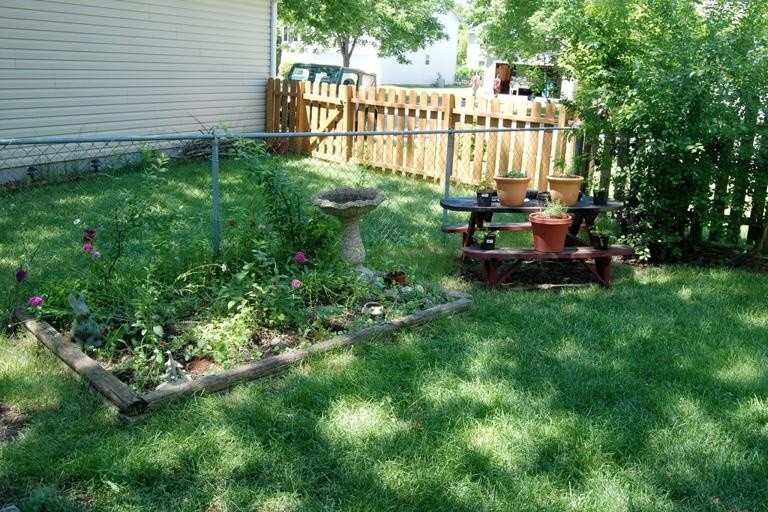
[493, 170, 529, 210]
[530, 200, 574, 253]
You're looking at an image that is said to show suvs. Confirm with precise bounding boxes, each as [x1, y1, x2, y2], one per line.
[277, 64, 379, 142]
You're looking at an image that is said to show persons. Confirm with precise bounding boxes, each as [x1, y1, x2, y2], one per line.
[492, 74, 502, 101]
[467, 71, 482, 98]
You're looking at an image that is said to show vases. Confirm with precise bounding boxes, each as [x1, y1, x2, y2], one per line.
[546, 171, 586, 208]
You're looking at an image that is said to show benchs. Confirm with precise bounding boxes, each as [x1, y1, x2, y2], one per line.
[458, 242, 637, 290]
[439, 217, 609, 258]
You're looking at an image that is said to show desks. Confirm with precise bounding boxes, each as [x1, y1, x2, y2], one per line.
[435, 194, 634, 258]
